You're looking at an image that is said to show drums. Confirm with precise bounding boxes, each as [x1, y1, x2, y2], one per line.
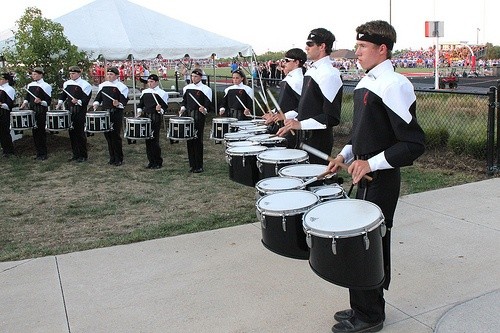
[255, 176, 307, 201]
[45, 110, 72, 131]
[312, 186, 345, 203]
[9, 110, 37, 131]
[224, 145, 267, 188]
[166, 117, 195, 141]
[84, 110, 112, 133]
[302, 198, 387, 291]
[256, 148, 310, 181]
[277, 163, 342, 188]
[223, 119, 289, 148]
[210, 117, 239, 141]
[124, 116, 152, 140]
[255, 189, 320, 261]
[225, 141, 262, 149]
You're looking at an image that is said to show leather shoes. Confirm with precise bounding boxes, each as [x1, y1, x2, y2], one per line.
[332, 319, 383, 333]
[334, 309, 355, 322]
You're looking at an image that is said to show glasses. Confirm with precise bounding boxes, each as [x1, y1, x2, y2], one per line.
[306, 42, 327, 47]
[285, 58, 302, 62]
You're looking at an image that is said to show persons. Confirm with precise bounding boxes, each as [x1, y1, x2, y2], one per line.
[326, 21, 426, 333]
[179, 68, 212, 173]
[0, 73, 15, 157]
[275, 28, 344, 165]
[261, 58, 288, 124]
[219, 70, 256, 148]
[264, 48, 307, 149]
[20, 67, 52, 161]
[136, 74, 169, 169]
[93, 67, 129, 166]
[55, 66, 92, 163]
[90, 46, 500, 89]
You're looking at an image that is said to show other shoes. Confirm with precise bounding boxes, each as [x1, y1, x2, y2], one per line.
[39, 156, 47, 160]
[116, 161, 122, 166]
[188, 167, 193, 173]
[77, 157, 86, 162]
[193, 168, 203, 172]
[148, 163, 162, 168]
[34, 156, 39, 159]
[108, 160, 115, 164]
[69, 157, 77, 161]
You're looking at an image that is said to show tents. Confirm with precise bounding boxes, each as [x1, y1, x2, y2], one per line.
[0, 0, 270, 120]
